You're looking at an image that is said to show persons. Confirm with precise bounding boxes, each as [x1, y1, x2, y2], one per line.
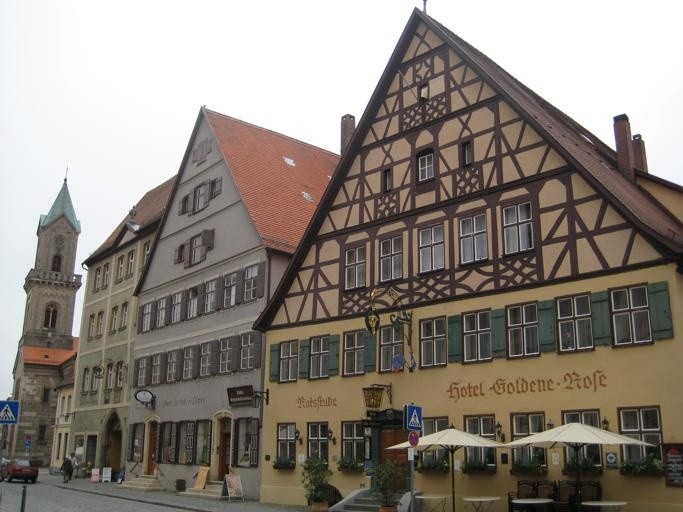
[61, 452, 78, 483]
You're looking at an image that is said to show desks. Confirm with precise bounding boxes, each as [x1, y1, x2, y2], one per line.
[415, 494, 501, 512]
[581, 500, 627, 512]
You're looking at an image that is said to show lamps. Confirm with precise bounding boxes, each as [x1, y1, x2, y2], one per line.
[362, 382, 392, 408]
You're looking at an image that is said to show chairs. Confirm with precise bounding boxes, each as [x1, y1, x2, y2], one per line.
[508, 480, 601, 512]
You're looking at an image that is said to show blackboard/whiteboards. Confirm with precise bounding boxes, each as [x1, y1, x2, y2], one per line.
[662, 444, 683, 487]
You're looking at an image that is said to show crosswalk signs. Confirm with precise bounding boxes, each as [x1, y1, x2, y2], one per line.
[403, 403, 424, 431]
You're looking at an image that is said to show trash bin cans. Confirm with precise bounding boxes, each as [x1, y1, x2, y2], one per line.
[176, 480, 186, 492]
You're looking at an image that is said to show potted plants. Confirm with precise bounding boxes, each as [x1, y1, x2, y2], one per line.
[271, 456, 412, 512]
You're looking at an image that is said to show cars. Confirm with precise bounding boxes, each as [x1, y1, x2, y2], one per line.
[0, 457, 39, 482]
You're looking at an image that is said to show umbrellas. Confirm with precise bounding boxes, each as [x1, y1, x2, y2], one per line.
[385, 421, 657, 512]
